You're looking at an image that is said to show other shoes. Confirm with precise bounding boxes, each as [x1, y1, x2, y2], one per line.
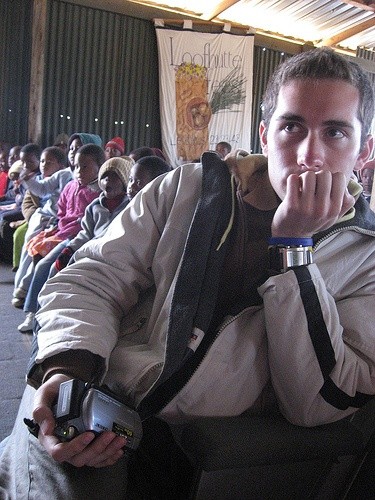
[13, 288, 27, 298]
[11, 298, 25, 307]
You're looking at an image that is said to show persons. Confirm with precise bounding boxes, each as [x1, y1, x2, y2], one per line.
[0, 46, 375, 500]
[0, 132, 375, 334]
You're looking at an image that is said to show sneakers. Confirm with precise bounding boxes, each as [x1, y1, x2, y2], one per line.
[17, 311, 37, 331]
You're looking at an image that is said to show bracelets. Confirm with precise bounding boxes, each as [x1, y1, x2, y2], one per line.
[267, 236, 314, 247]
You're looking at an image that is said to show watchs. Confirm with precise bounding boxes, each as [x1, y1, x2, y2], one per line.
[267, 244, 315, 273]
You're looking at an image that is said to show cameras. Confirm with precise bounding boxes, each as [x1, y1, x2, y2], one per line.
[51, 378, 143, 457]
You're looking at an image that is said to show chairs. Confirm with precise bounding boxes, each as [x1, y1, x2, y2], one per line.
[183, 397, 375, 500]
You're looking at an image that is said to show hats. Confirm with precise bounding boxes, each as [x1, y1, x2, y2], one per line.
[105, 137, 125, 156]
[8, 160, 24, 180]
[360, 158, 375, 179]
[98, 156, 135, 190]
